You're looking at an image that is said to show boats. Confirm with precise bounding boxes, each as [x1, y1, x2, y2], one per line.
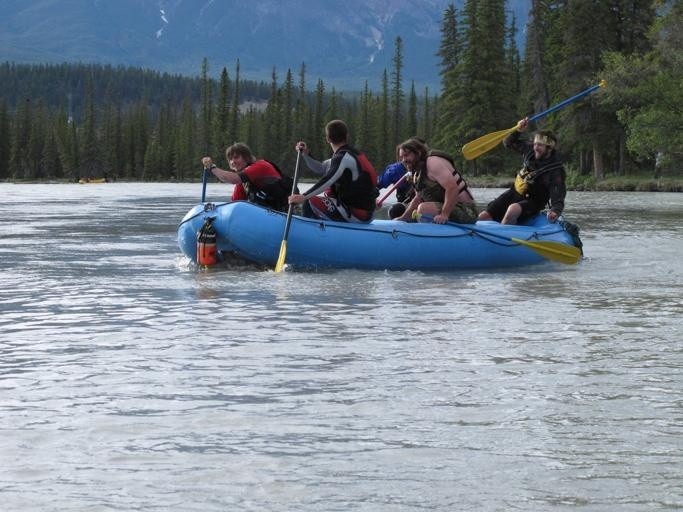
[177, 200, 584, 271]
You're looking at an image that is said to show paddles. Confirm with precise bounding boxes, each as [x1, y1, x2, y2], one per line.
[274, 142, 305, 273]
[462, 79, 606, 161]
[411, 209, 581, 264]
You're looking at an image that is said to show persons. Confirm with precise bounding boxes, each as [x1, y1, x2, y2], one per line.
[283, 119, 380, 223]
[202, 141, 292, 213]
[375, 137, 430, 223]
[390, 139, 478, 225]
[478, 116, 569, 226]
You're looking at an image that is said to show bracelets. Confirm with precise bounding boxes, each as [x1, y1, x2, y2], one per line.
[206, 163, 217, 171]
